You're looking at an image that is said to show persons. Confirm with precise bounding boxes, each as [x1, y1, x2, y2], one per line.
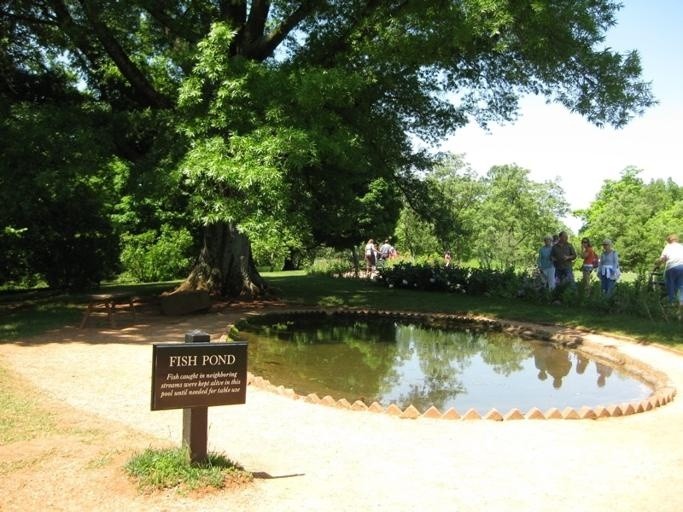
[531, 344, 551, 380]
[576, 356, 589, 373]
[595, 362, 613, 386]
[363, 238, 397, 277]
[653, 234, 683, 308]
[578, 236, 595, 291]
[537, 231, 577, 294]
[546, 349, 571, 388]
[596, 239, 620, 294]
[444, 250, 452, 268]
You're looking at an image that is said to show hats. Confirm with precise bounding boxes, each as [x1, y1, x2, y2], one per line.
[599, 239, 611, 245]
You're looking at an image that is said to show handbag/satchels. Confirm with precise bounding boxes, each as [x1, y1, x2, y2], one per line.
[591, 251, 597, 267]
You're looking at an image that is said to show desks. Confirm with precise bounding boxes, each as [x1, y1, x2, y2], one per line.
[78, 291, 138, 329]
